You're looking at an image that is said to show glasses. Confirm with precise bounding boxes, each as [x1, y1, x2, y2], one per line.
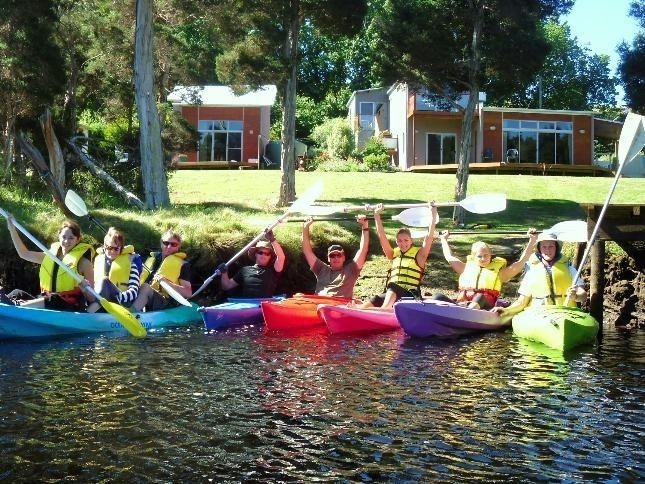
[162, 240, 179, 247]
[257, 250, 271, 256]
[105, 244, 121, 252]
[329, 253, 343, 258]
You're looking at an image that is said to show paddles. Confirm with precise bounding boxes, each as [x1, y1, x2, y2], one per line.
[287, 193, 506, 214]
[65, 189, 193, 308]
[0, 208, 147, 337]
[563, 111, 645, 308]
[242, 207, 440, 228]
[402, 220, 588, 243]
[185, 181, 323, 301]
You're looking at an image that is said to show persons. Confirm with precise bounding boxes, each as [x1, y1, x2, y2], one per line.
[4, 209, 95, 313]
[490, 228, 588, 316]
[439, 223, 541, 311]
[303, 212, 369, 296]
[125, 224, 193, 312]
[215, 225, 286, 299]
[85, 225, 143, 313]
[356, 200, 444, 311]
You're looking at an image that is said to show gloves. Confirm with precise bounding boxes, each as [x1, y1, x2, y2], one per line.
[264, 228, 276, 242]
[217, 263, 227, 273]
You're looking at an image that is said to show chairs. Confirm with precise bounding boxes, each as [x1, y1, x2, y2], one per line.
[506, 149, 518, 163]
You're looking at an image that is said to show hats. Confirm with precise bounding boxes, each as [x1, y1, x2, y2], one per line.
[327, 244, 344, 256]
[534, 232, 564, 253]
[247, 238, 274, 256]
